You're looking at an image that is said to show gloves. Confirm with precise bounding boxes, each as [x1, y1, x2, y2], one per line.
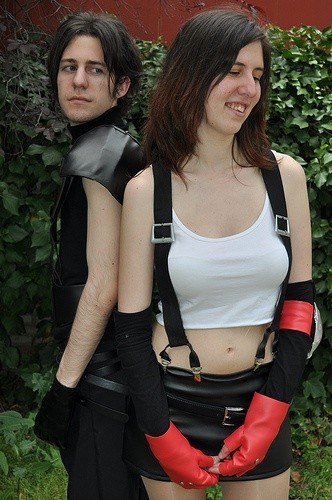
[33, 374, 74, 449]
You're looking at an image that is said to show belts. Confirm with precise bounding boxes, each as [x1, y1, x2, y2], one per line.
[166, 386, 249, 429]
[76, 359, 133, 421]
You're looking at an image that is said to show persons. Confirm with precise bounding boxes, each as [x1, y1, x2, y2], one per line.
[30, 12, 151, 500]
[118, 11, 322, 500]
